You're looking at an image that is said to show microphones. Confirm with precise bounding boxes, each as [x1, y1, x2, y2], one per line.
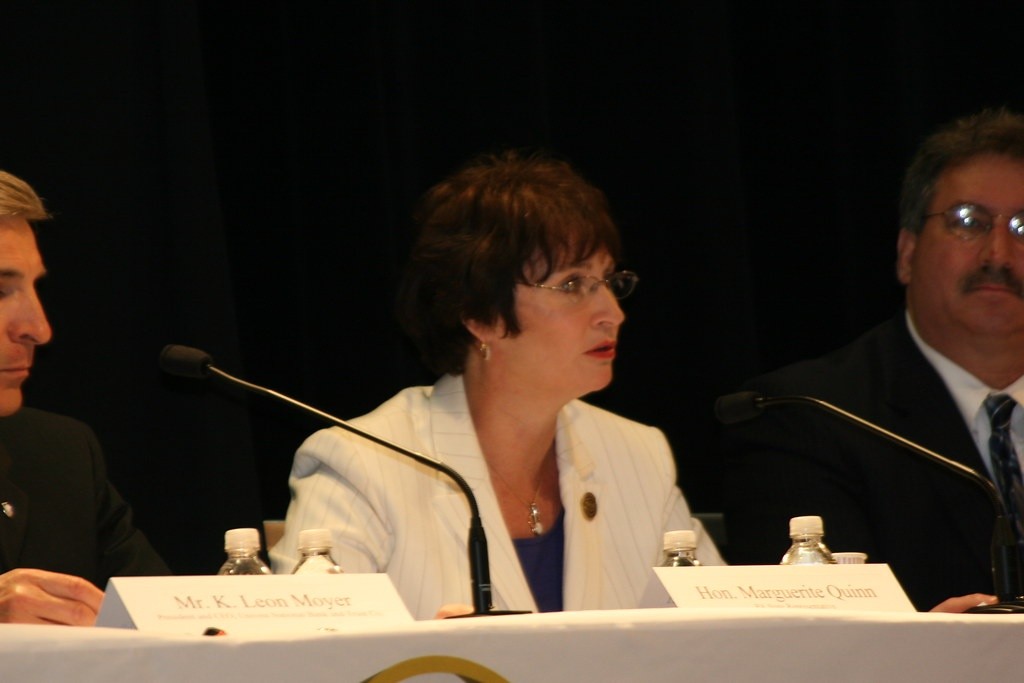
[712, 389, 1024, 614]
[160, 343, 494, 615]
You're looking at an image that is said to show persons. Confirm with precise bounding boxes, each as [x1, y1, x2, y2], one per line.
[0, 169, 173, 630]
[717, 113, 1023, 613]
[268, 152, 727, 623]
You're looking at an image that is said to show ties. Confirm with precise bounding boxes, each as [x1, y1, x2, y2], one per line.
[983, 392, 1024, 543]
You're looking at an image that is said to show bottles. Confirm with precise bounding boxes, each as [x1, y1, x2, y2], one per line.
[778, 516, 839, 565]
[661, 530, 704, 567]
[216, 528, 273, 575]
[289, 528, 343, 574]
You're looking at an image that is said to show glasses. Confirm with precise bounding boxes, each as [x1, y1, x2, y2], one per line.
[922, 197, 1024, 242]
[517, 269, 638, 310]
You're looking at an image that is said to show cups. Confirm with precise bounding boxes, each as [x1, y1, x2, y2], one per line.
[830, 552, 867, 564]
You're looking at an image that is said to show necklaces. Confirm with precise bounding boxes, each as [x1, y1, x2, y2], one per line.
[484, 455, 548, 537]
